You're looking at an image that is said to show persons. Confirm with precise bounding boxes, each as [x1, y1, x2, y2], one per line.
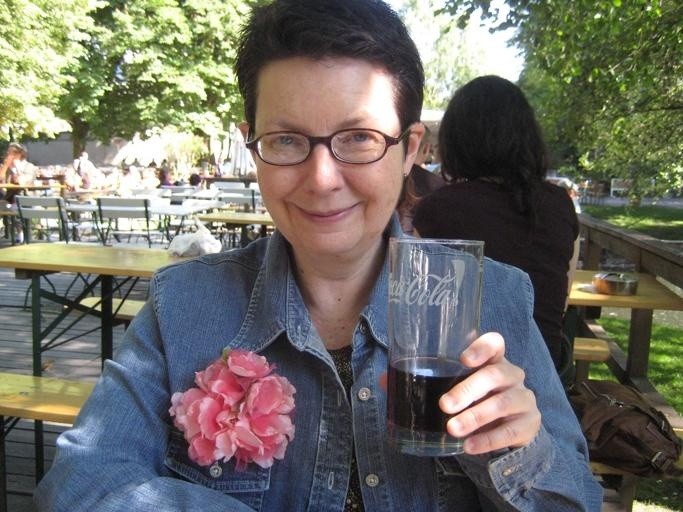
[412, 75, 581, 390]
[33, 0, 603, 512]
[0, 142, 234, 244]
[396, 125, 446, 235]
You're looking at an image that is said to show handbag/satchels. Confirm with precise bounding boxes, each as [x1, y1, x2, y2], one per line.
[567, 378, 682, 476]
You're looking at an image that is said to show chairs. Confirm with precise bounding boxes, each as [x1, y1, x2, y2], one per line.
[13, 183, 256, 248]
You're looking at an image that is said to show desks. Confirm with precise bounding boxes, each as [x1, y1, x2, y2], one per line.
[565, 269, 680, 488]
[1, 208, 279, 384]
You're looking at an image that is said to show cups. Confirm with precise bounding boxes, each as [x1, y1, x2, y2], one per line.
[385, 235, 487, 461]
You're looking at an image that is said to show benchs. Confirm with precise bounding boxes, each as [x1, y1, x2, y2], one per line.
[0, 210, 17, 245]
[0, 369, 638, 510]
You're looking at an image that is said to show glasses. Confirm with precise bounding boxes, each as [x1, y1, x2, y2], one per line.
[246, 125, 411, 164]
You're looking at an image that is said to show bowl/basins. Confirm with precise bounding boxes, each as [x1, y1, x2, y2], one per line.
[593, 272, 640, 295]
